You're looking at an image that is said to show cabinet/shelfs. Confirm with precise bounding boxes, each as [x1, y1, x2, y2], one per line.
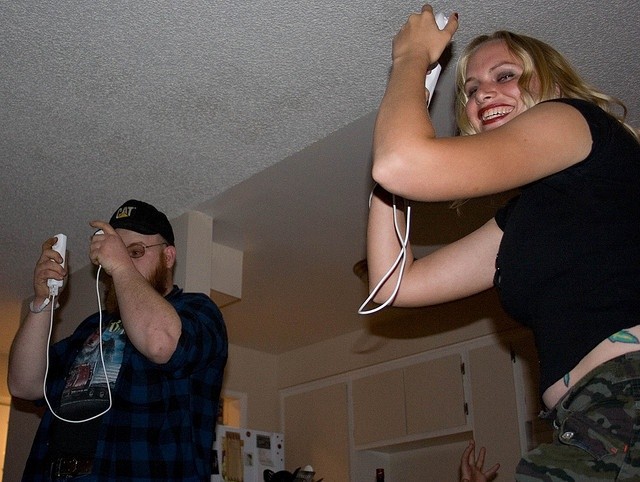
[278, 373, 349, 481]
[467, 332, 535, 481]
[348, 341, 472, 452]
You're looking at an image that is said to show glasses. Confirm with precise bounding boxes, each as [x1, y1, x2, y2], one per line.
[124, 242, 169, 258]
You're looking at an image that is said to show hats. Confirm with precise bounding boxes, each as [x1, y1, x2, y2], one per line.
[109, 199, 174, 245]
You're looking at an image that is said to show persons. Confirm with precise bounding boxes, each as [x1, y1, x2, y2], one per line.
[460, 438, 500, 482]
[7, 199, 228, 481]
[367, 5, 640, 482]
